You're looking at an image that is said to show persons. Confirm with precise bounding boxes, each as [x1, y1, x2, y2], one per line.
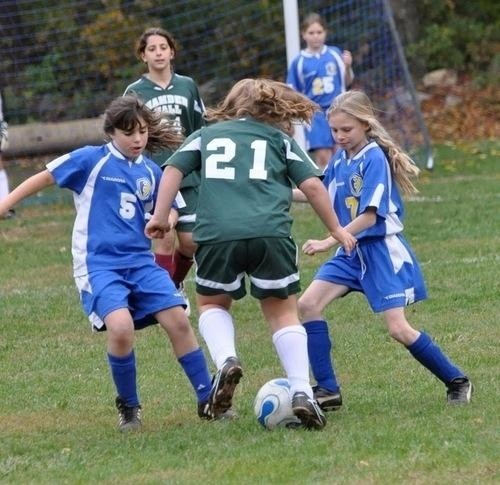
[0, 97, 16, 222]
[290, 86, 476, 413]
[283, 11, 356, 174]
[143, 76, 361, 433]
[1, 92, 242, 434]
[122, 27, 213, 318]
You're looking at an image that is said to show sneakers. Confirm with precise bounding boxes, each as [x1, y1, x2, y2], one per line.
[177, 280, 192, 318]
[115, 401, 143, 430]
[290, 390, 328, 432]
[198, 400, 240, 422]
[206, 356, 243, 419]
[312, 385, 343, 413]
[445, 375, 474, 408]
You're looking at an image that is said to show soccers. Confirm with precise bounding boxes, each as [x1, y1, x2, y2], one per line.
[254, 378, 302, 431]
